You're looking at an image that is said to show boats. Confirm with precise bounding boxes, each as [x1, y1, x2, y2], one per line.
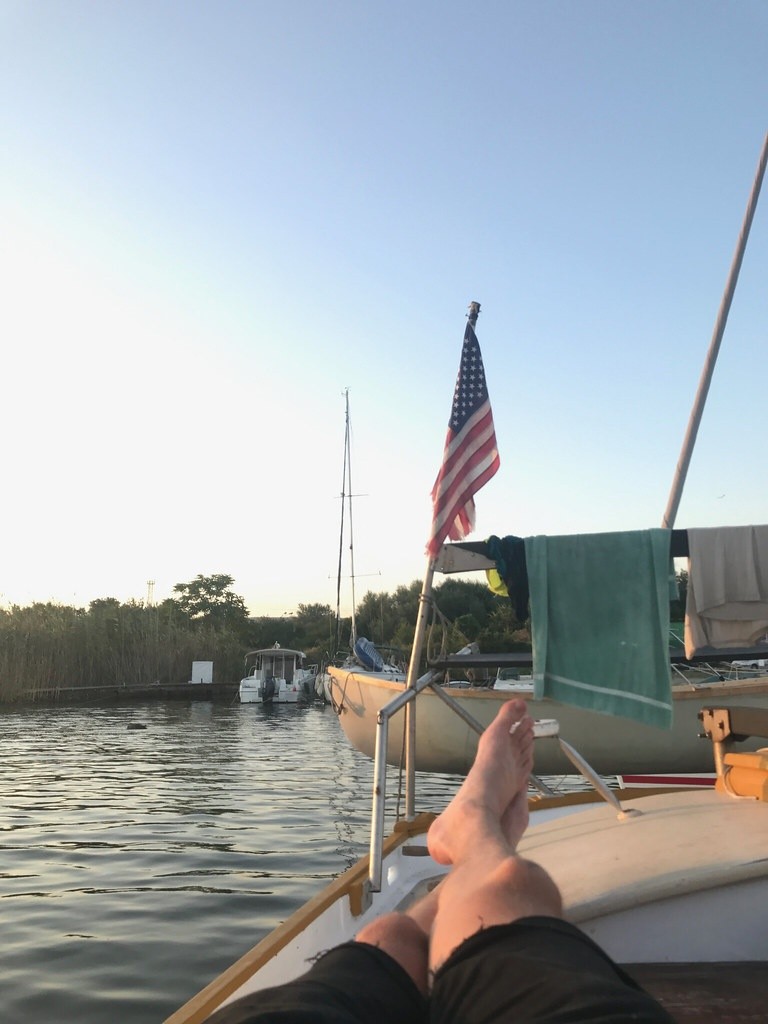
[325, 137, 768, 776]
[240, 642, 313, 703]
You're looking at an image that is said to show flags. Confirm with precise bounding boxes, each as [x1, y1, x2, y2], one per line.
[423, 321, 501, 553]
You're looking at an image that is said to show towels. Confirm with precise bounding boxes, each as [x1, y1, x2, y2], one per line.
[683, 524, 768, 662]
[485, 527, 676, 733]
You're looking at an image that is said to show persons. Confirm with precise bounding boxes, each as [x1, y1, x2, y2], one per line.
[204, 694, 675, 1024]
